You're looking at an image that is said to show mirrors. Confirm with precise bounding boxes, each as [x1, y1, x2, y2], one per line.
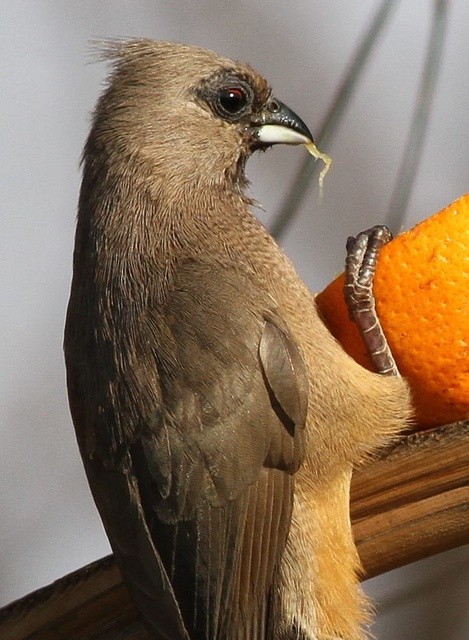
[63, 34, 418, 640]
